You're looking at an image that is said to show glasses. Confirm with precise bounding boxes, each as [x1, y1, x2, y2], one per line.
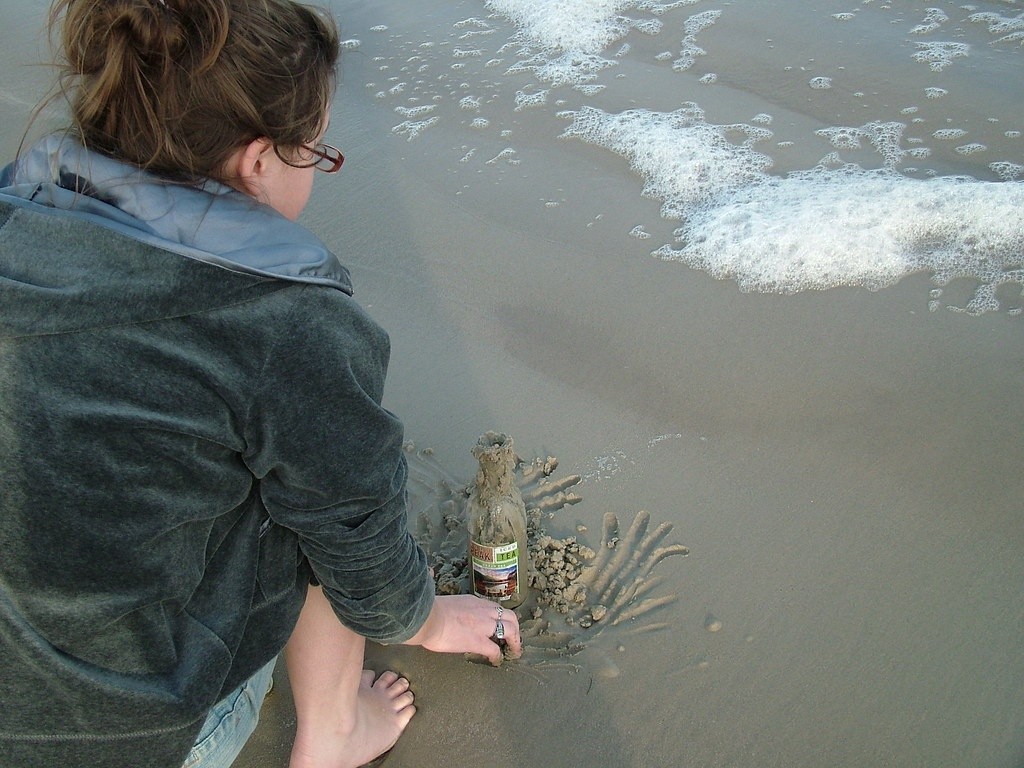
[281, 139, 344, 173]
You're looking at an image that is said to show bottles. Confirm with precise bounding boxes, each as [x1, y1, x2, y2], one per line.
[466, 434, 529, 609]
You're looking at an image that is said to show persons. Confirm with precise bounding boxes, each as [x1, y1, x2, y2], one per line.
[0, 0, 524, 767]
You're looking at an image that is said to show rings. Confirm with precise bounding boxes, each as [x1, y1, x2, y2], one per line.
[494, 622, 504, 639]
[495, 606, 503, 618]
